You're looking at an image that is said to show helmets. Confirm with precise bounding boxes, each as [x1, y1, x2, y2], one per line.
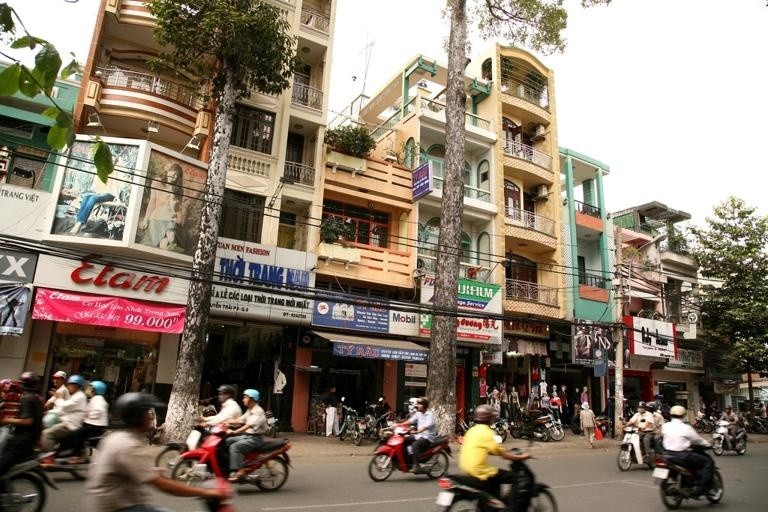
[669, 405, 686, 416]
[473, 404, 497, 424]
[215, 384, 235, 398]
[21, 370, 106, 396]
[416, 398, 429, 412]
[110, 391, 169, 431]
[243, 389, 258, 402]
[637, 400, 645, 409]
[646, 400, 658, 411]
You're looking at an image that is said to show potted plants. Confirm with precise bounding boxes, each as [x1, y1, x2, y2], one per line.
[317, 216, 362, 265]
[326, 126, 377, 174]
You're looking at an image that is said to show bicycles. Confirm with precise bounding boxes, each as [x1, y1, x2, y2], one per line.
[453, 408, 508, 443]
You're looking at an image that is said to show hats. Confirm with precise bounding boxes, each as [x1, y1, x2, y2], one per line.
[581, 402, 589, 409]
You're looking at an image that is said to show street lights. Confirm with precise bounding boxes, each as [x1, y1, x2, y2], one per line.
[626, 232, 668, 303]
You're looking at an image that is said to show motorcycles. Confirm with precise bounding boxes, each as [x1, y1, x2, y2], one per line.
[693, 415, 715, 432]
[753, 416, 768, 434]
[652, 445, 724, 509]
[507, 396, 565, 443]
[616, 416, 663, 471]
[154, 396, 292, 498]
[710, 417, 750, 456]
[196, 478, 244, 512]
[434, 449, 558, 512]
[37, 430, 114, 481]
[337, 395, 454, 481]
[0, 401, 61, 512]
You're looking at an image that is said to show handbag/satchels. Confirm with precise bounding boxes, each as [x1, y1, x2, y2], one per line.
[594, 427, 603, 440]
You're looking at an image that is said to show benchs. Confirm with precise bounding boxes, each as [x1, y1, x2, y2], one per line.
[75, 198, 126, 238]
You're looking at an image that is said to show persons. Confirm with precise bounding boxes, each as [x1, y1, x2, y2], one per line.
[553, 385, 570, 426]
[1, 296, 24, 327]
[69, 154, 124, 236]
[740, 408, 745, 420]
[322, 384, 342, 437]
[756, 402, 766, 420]
[485, 385, 521, 425]
[654, 394, 662, 409]
[580, 402, 598, 449]
[139, 163, 184, 245]
[375, 396, 392, 443]
[0, 370, 270, 511]
[662, 405, 716, 496]
[533, 390, 563, 421]
[398, 397, 436, 472]
[273, 370, 287, 422]
[459, 404, 531, 508]
[620, 400, 664, 459]
[697, 399, 739, 449]
[574, 319, 630, 369]
[571, 385, 588, 423]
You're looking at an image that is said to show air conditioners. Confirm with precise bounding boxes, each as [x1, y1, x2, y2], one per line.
[529, 124, 546, 142]
[532, 185, 548, 202]
